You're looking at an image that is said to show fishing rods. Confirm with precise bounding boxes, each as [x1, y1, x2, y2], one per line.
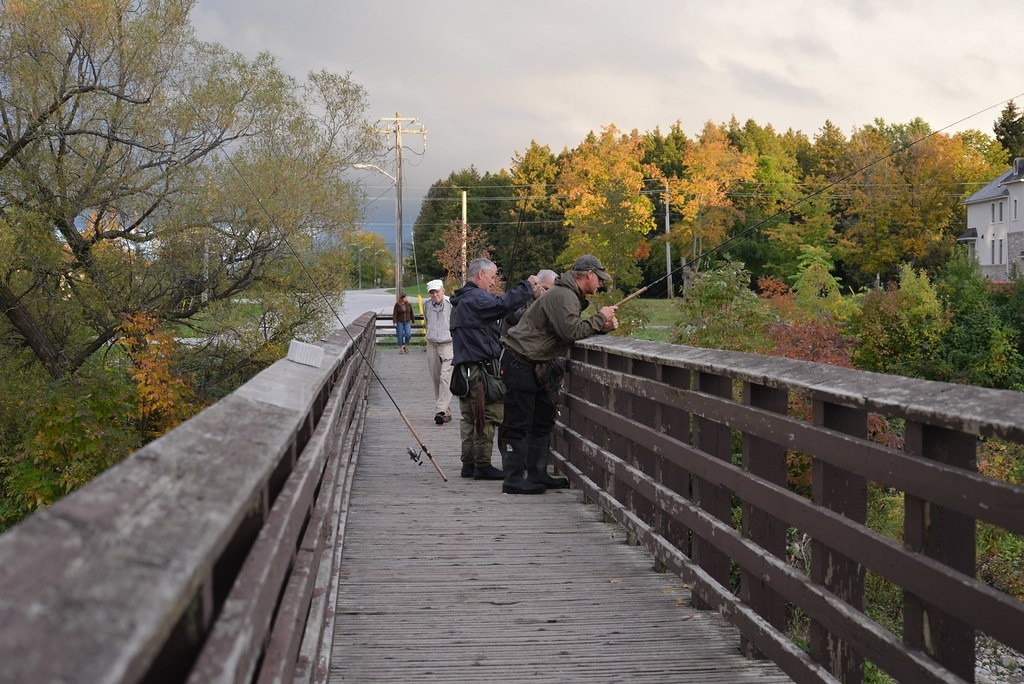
[496, 198, 528, 330]
[614, 90, 1024, 310]
[132, 24, 448, 481]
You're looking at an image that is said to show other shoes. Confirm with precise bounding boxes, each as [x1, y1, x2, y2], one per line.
[434, 414, 452, 424]
[461, 463, 505, 480]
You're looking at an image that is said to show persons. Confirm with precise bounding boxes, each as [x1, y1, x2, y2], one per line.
[450, 259, 538, 480]
[422, 279, 453, 424]
[497, 256, 617, 493]
[393, 293, 416, 354]
[498, 269, 557, 467]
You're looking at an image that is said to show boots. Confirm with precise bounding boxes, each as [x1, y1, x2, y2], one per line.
[498, 434, 569, 494]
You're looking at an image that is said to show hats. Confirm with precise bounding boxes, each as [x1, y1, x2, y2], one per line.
[426, 280, 443, 293]
[572, 253, 610, 280]
